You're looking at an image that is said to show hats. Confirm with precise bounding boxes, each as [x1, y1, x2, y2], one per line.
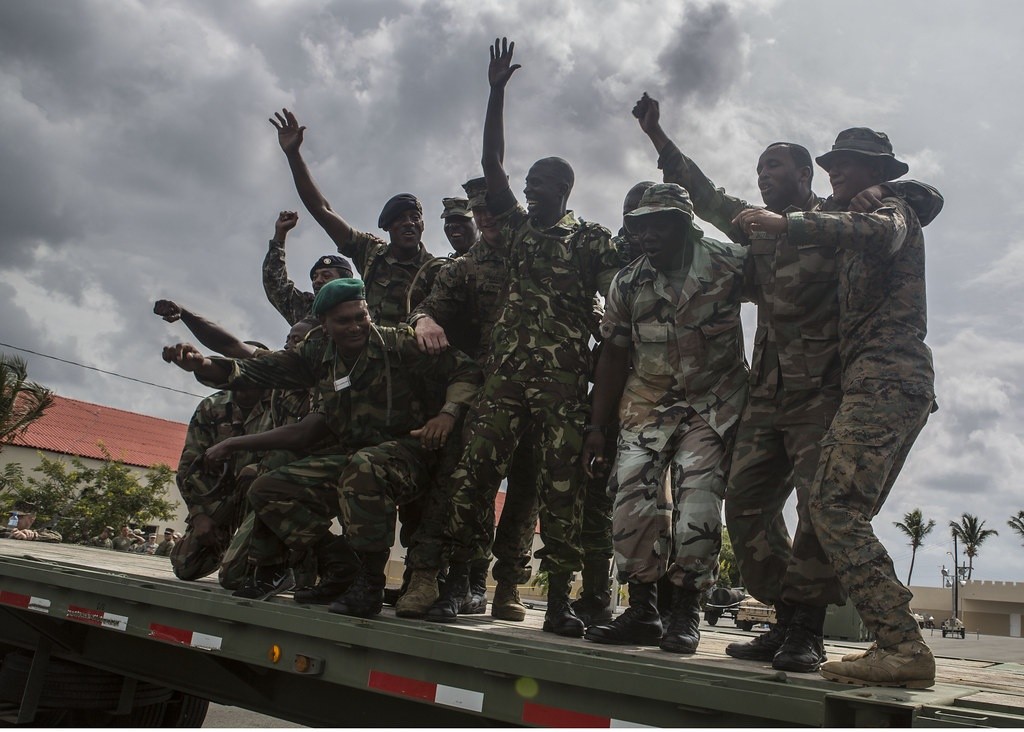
[104, 525, 183, 542]
[6, 500, 39, 516]
[815, 127, 910, 183]
[624, 183, 704, 243]
[440, 196, 474, 219]
[310, 255, 353, 281]
[461, 175, 510, 210]
[377, 192, 423, 232]
[312, 278, 366, 316]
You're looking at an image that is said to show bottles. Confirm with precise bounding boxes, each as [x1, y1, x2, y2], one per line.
[5, 511, 18, 539]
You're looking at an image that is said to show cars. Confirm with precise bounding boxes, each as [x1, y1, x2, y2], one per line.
[942, 617, 965, 639]
[701, 588, 777, 632]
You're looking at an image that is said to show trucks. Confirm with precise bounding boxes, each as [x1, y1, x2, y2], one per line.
[3, 537, 1022, 728]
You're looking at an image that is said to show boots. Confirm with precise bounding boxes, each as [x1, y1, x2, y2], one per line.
[327, 553, 387, 617]
[570, 569, 613, 626]
[658, 586, 702, 654]
[542, 571, 585, 638]
[725, 602, 828, 673]
[584, 581, 664, 647]
[392, 559, 490, 624]
[293, 531, 362, 604]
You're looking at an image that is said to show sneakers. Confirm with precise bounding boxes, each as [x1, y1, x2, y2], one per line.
[231, 566, 296, 603]
[819, 640, 936, 689]
[491, 581, 526, 621]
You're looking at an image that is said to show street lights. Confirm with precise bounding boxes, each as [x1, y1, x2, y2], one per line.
[941, 536, 969, 618]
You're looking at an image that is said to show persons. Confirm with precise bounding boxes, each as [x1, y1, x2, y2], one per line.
[159, 274, 486, 620]
[87, 525, 182, 559]
[383, 195, 490, 613]
[261, 210, 356, 324]
[270, 107, 444, 607]
[0, 500, 63, 543]
[431, 34, 621, 637]
[730, 126, 937, 688]
[154, 299, 317, 596]
[577, 183, 752, 653]
[171, 338, 274, 579]
[415, 172, 541, 621]
[572, 183, 660, 625]
[630, 88, 946, 674]
